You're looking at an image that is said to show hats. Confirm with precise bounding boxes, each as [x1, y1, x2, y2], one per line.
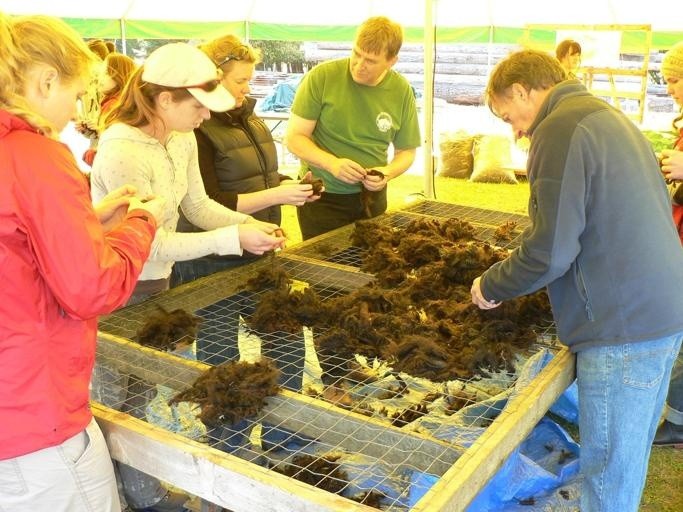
[661, 40, 682, 79]
[140, 42, 235, 113]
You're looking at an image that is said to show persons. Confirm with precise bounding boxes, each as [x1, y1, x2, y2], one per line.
[0, 16, 169, 511]
[650, 47, 683, 247]
[166, 36, 322, 455]
[280, 23, 423, 390]
[88, 39, 292, 512]
[62, 36, 135, 175]
[463, 48, 683, 512]
[554, 38, 586, 72]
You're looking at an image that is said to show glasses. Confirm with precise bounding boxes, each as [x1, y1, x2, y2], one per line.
[217, 45, 248, 67]
[165, 67, 223, 92]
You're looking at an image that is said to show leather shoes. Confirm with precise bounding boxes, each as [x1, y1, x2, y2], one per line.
[346, 360, 377, 384]
[652, 419, 682, 449]
[322, 381, 351, 408]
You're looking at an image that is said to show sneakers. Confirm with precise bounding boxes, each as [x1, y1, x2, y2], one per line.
[146, 489, 191, 512]
[235, 443, 266, 466]
